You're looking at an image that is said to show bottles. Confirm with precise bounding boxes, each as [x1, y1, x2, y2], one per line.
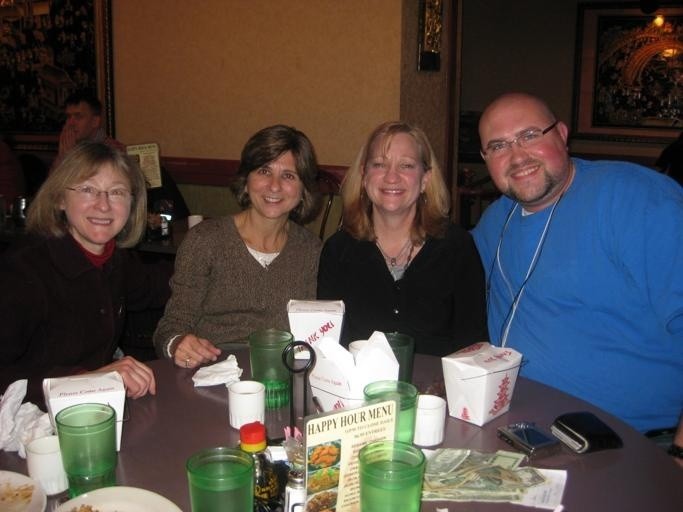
[236, 422, 280, 511]
[285, 469, 307, 508]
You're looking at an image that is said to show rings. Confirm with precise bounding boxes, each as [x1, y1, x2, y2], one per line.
[185, 356, 192, 368]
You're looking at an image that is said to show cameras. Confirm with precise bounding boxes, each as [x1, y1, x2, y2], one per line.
[497, 421, 562, 458]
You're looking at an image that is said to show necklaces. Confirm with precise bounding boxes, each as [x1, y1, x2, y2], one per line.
[375, 238, 417, 267]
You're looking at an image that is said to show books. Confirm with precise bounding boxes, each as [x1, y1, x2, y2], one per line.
[303, 396, 397, 512]
[126, 141, 162, 189]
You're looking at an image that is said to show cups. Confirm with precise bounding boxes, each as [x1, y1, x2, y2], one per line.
[54, 402, 119, 499]
[186, 447, 255, 512]
[227, 381, 267, 430]
[187, 215, 203, 229]
[247, 328, 295, 442]
[357, 439, 426, 512]
[362, 379, 420, 447]
[385, 332, 417, 383]
[24, 434, 69, 497]
[413, 393, 448, 448]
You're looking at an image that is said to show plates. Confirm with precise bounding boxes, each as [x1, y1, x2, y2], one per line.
[49, 485, 185, 512]
[1, 467, 49, 512]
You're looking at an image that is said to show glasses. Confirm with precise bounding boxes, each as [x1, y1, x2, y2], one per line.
[65, 183, 140, 200]
[484, 122, 561, 156]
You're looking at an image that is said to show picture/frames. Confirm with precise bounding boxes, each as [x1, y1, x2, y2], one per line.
[571, 0, 683, 145]
[0, 0, 115, 152]
[418, 2, 445, 73]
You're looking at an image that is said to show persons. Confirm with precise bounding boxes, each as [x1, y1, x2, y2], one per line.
[318, 119, 490, 357]
[1, 136, 156, 402]
[49, 92, 127, 172]
[469, 91, 683, 474]
[152, 123, 326, 367]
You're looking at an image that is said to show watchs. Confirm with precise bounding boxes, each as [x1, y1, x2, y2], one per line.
[667, 442, 683, 461]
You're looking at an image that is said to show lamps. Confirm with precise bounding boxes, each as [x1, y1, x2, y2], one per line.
[652, 12, 665, 30]
